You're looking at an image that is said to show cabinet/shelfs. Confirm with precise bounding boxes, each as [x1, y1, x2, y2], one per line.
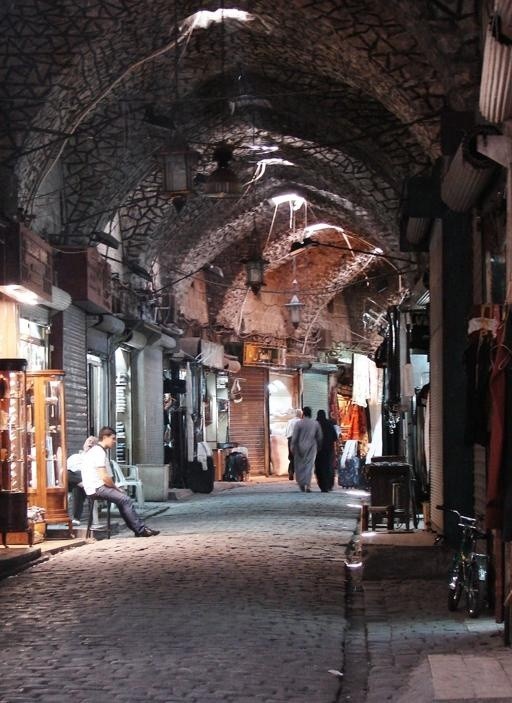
[0, 358, 73, 549]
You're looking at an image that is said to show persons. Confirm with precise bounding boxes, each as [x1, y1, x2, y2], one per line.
[65, 436, 107, 526]
[80, 425, 160, 538]
[314, 408, 338, 492]
[290, 405, 324, 492]
[284, 408, 304, 481]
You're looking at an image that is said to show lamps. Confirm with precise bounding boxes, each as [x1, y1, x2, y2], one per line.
[240, 103, 268, 297]
[288, 238, 417, 266]
[283, 254, 304, 330]
[152, 1, 199, 217]
[206, 1, 243, 199]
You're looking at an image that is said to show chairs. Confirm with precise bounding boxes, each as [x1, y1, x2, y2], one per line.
[110, 459, 144, 509]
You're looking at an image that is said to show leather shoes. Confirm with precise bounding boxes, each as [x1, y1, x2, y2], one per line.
[135, 526, 159, 537]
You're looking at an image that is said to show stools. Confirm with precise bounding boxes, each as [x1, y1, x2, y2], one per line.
[85, 496, 112, 538]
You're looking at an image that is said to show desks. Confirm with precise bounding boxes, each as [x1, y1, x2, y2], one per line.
[372, 510, 412, 531]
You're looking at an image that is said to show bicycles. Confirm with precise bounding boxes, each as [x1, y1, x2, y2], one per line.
[436, 504, 490, 618]
